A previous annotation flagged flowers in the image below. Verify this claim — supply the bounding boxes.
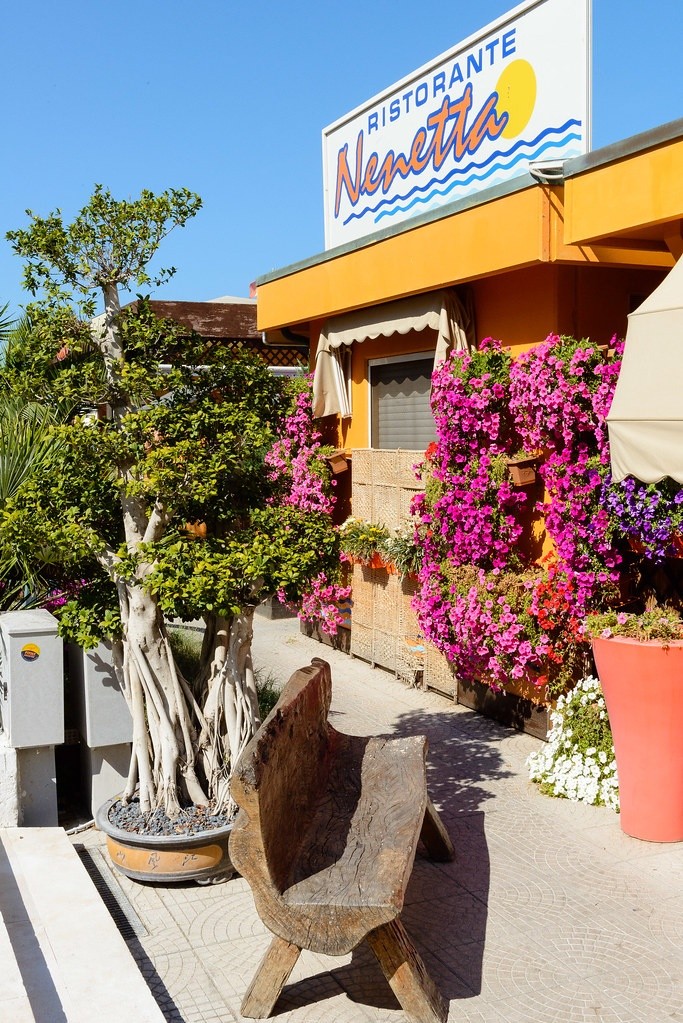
[339,515,389,564]
[411,332,683,691]
[262,366,352,636]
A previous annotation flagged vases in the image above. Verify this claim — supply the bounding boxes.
[587,632,682,842]
[356,553,386,569]
[506,454,537,485]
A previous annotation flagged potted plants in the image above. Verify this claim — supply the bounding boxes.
[2,186,342,887]
[384,529,421,582]
[320,444,348,474]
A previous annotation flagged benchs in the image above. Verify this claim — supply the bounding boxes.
[227,658,456,1023]
